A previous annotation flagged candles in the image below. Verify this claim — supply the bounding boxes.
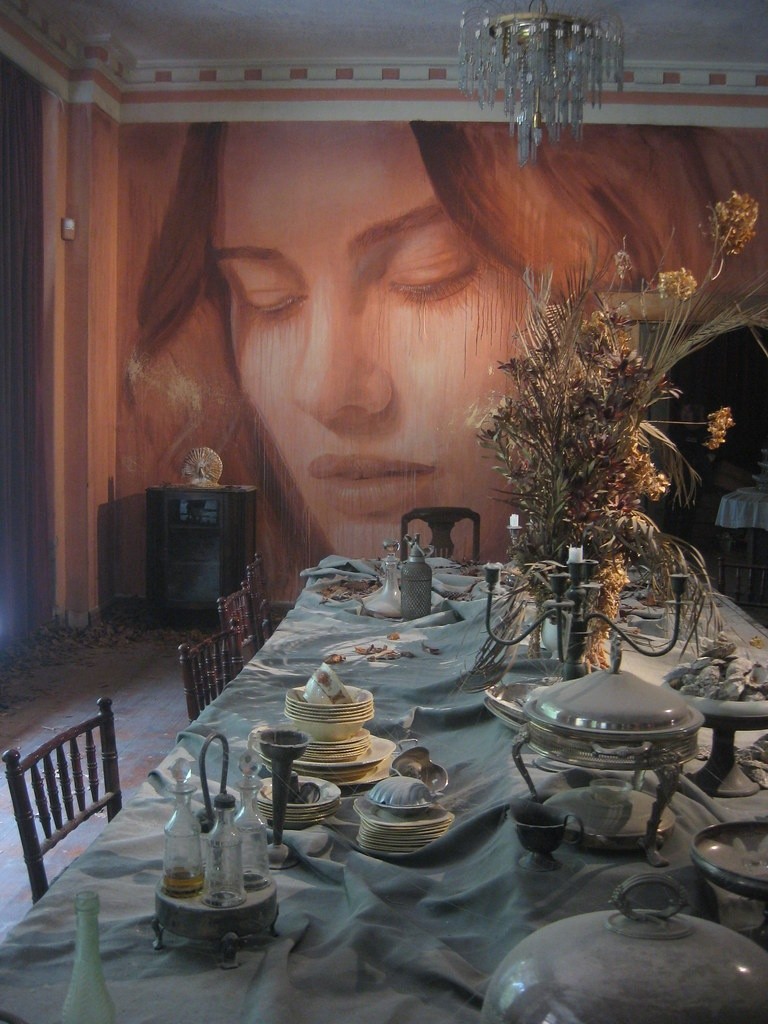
[510,514,519,528]
[567,543,584,562]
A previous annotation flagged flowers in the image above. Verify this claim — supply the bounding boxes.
[452,191,759,694]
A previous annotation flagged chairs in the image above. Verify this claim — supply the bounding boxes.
[717,556,768,630]
[216,580,259,671]
[1,696,122,906]
[399,507,481,565]
[178,618,244,723]
[246,550,273,654]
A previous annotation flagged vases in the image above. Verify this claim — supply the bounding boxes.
[542,608,573,660]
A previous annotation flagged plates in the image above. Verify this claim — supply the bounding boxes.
[352,798,455,854]
[236,728,396,787]
[256,774,341,830]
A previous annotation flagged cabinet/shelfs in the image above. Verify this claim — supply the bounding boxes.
[145,482,258,626]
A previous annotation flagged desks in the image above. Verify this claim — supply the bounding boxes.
[714,487,768,566]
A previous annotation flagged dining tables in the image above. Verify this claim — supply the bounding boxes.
[1,555,768,1024]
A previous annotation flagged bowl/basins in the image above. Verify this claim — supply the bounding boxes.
[282,686,375,741]
[590,776,633,805]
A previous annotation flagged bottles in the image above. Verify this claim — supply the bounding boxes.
[160,783,204,898]
[365,538,402,620]
[202,790,247,908]
[61,891,115,1024]
[401,533,432,618]
[233,775,271,893]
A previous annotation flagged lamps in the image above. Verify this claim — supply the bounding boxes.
[455,0,626,170]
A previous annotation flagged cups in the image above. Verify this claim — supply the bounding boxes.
[505,799,583,873]
